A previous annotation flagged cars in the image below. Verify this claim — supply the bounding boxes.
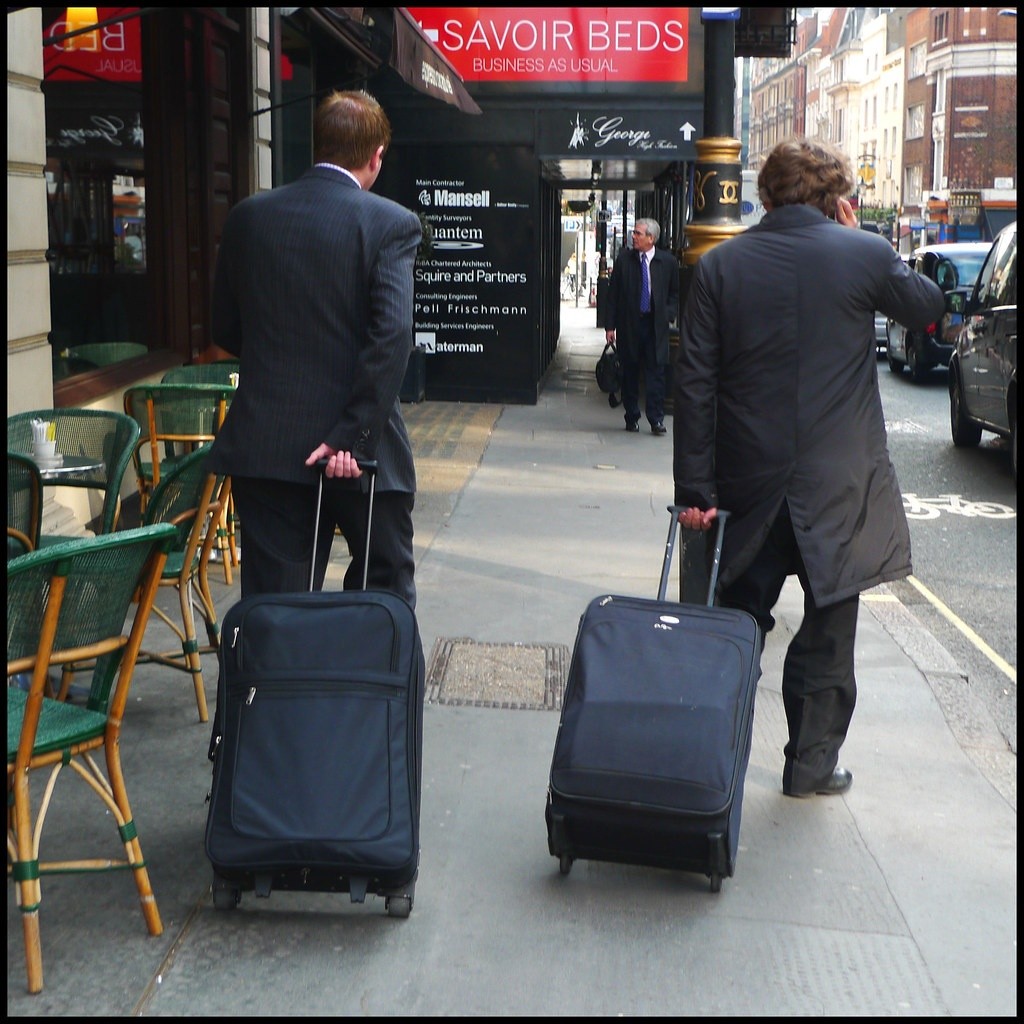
[874,243,994,377]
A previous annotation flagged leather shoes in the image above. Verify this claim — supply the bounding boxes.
[651,422,666,433]
[626,420,639,431]
[788,766,851,798]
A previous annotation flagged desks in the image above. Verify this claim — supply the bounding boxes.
[133,399,244,564]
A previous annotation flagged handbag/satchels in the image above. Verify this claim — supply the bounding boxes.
[596,342,625,409]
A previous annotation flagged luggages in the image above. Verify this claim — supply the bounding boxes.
[203,455,424,918]
[545,505,760,892]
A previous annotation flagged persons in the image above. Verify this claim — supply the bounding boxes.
[604,218,681,433]
[670,140,947,799]
[203,89,423,604]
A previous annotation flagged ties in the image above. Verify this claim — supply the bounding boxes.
[640,252,650,314]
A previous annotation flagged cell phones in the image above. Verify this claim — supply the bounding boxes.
[828,209,837,222]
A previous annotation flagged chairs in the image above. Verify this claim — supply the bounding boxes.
[7,407,140,553]
[5,450,59,701]
[159,358,241,406]
[55,443,221,724]
[0,522,178,994]
[123,383,239,586]
[62,342,147,371]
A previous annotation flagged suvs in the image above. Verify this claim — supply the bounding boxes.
[945,222,1017,480]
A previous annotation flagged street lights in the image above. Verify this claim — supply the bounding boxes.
[856,176,868,229]
